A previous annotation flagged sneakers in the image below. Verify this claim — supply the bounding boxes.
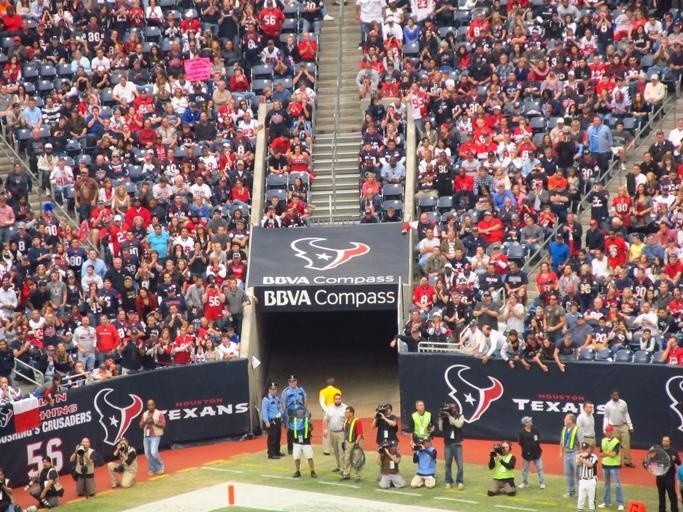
[563,491,578,498]
[519,484,528,488]
[598,503,611,509]
[333,468,360,483]
[540,484,546,488]
[445,481,463,491]
[292,472,300,477]
[311,470,316,479]
[269,452,285,459]
[617,506,623,510]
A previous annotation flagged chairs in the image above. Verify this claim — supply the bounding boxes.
[1,0,320,401]
[359,0,683,368]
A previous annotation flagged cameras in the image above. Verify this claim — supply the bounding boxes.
[120,442,126,452]
[77,445,85,457]
[41,498,53,509]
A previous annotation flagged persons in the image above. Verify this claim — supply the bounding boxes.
[261,377,364,482]
[642,435,681,511]
[107,439,137,488]
[0,1,334,404]
[1,438,96,511]
[356,1,681,371]
[372,400,464,489]
[139,399,166,475]
[488,416,546,496]
[559,392,635,510]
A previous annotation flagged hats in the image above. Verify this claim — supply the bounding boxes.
[521,417,531,423]
[287,375,296,380]
[580,442,589,448]
[605,425,613,431]
[267,382,277,390]
